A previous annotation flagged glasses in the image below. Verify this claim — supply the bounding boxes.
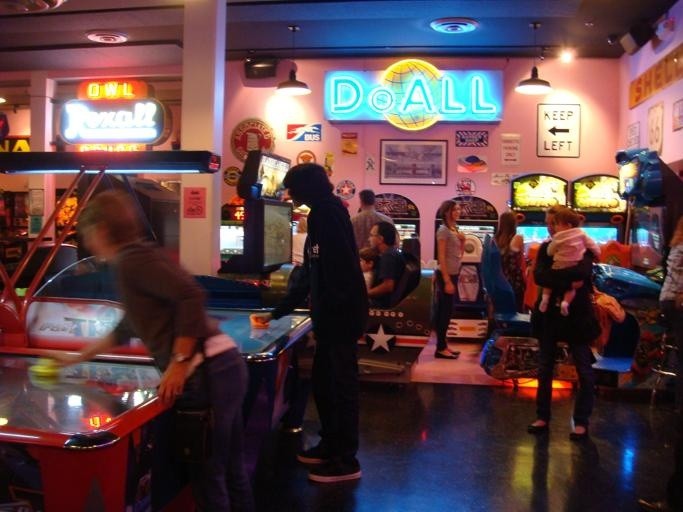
[369,234,378,237]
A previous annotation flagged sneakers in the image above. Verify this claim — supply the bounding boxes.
[298,439,322,464]
[309,458,361,482]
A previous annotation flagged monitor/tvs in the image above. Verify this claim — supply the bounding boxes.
[514,223,552,259]
[434,221,494,265]
[392,219,418,251]
[245,202,293,269]
[577,226,618,249]
[216,221,245,256]
[237,151,290,198]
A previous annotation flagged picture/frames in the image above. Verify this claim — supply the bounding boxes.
[380,139,447,186]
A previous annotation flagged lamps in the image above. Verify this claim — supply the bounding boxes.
[276,69,311,96]
[515,67,551,95]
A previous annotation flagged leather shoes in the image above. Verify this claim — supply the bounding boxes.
[570,426,589,439]
[528,422,548,432]
[450,351,460,354]
[435,351,458,359]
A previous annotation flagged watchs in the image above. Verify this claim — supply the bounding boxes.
[170,353,191,363]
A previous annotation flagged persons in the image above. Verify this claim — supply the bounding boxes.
[523,244,540,311]
[248,163,371,483]
[358,222,403,308]
[538,207,601,316]
[659,213,683,404]
[527,204,602,441]
[39,190,254,512]
[433,199,466,360]
[481,211,528,339]
[349,189,401,263]
